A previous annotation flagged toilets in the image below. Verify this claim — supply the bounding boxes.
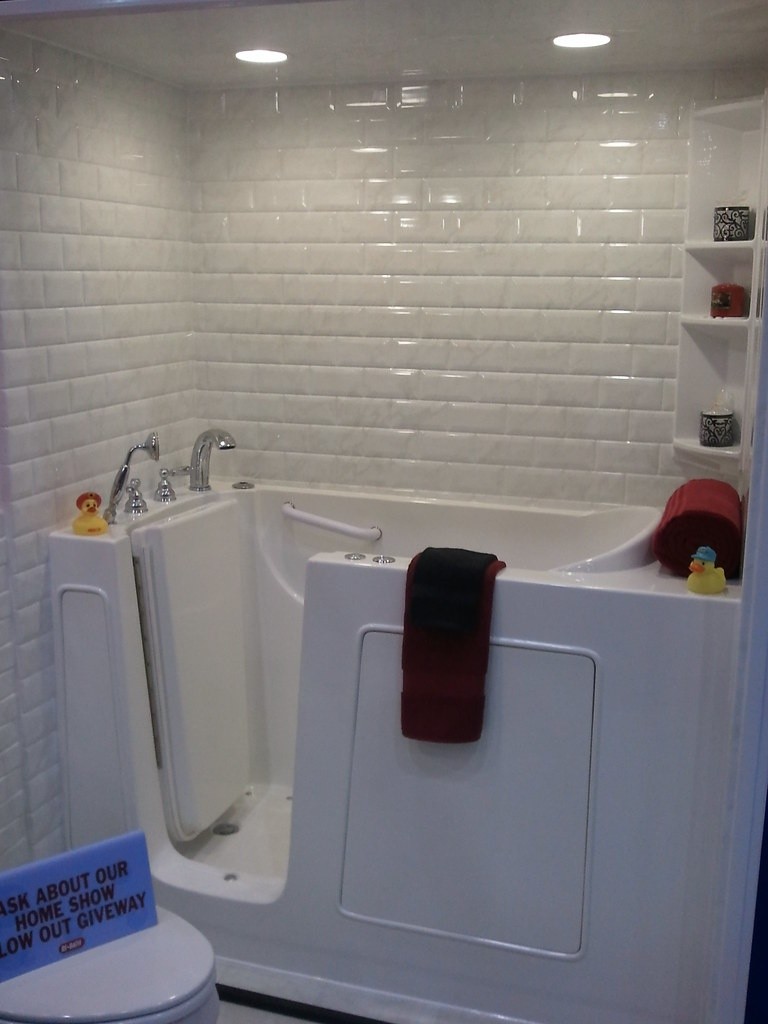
[0,904,219,1024]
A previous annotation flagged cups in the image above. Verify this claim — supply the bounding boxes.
[713,205,750,242]
[711,283,745,318]
[700,405,734,447]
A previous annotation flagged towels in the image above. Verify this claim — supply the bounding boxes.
[400,547,506,744]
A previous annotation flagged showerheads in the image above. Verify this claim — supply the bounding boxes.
[99,430,161,522]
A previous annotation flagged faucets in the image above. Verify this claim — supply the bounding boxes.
[189,429,236,491]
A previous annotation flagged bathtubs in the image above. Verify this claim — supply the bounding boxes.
[49,482,740,1024]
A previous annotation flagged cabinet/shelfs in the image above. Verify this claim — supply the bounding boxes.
[672,100,768,471]
